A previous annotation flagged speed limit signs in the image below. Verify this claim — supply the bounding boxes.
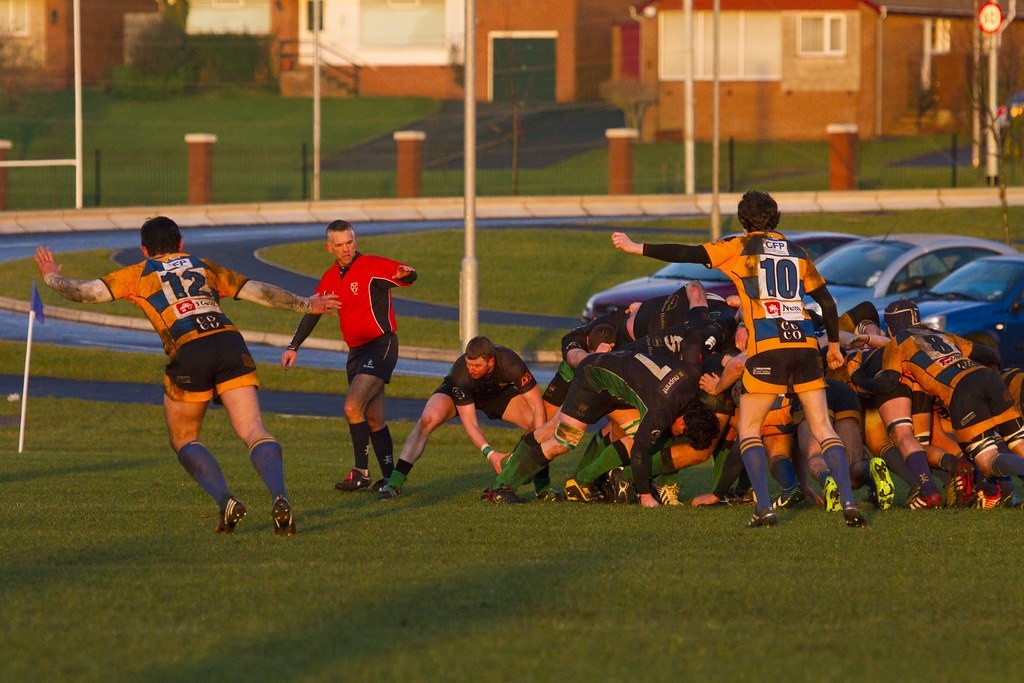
[976,1,1004,34]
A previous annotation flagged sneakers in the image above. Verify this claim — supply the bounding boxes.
[536,487,564,502]
[377,487,401,501]
[335,469,371,490]
[844,507,867,528]
[370,479,383,491]
[747,508,776,527]
[213,496,246,533]
[562,459,1022,510]
[272,496,295,536]
[493,488,520,505]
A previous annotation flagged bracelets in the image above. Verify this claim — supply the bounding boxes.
[480,443,494,459]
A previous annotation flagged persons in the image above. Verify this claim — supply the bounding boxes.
[377,337,566,503]
[612,188,866,528]
[281,221,417,491]
[487,281,1024,511]
[32,214,342,535]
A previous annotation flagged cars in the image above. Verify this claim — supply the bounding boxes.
[577,228,869,328]
[796,231,1024,332]
[880,253,1024,372]
